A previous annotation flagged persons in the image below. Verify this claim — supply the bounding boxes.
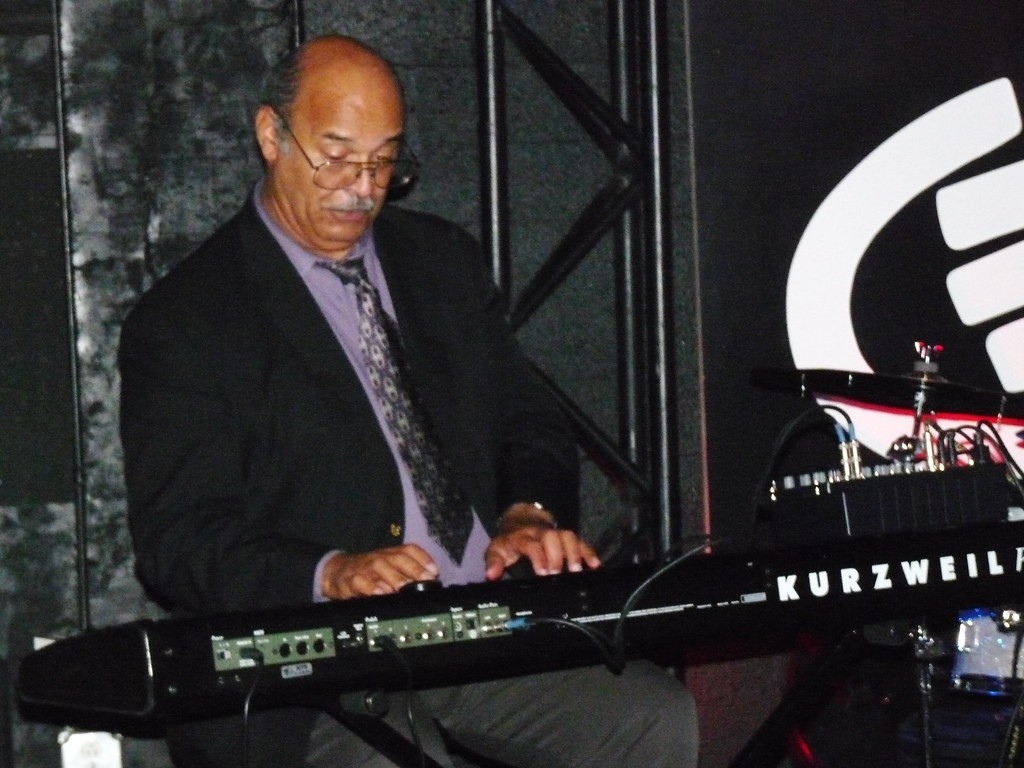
[118,33,700,768]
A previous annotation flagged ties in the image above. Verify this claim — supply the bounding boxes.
[317,255,474,566]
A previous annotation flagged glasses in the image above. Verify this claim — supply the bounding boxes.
[275,111,420,191]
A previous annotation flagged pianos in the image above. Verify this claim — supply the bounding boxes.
[10,517,1024,744]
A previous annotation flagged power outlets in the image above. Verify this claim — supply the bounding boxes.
[58,731,123,768]
